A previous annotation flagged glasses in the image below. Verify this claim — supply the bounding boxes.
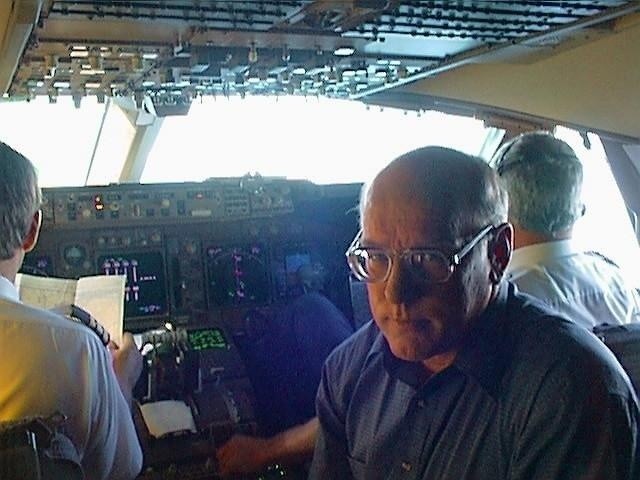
[345,223,496,287]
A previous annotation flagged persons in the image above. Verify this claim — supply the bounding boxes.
[211,126,639,478]
[0,140,148,480]
[304,143,638,479]
